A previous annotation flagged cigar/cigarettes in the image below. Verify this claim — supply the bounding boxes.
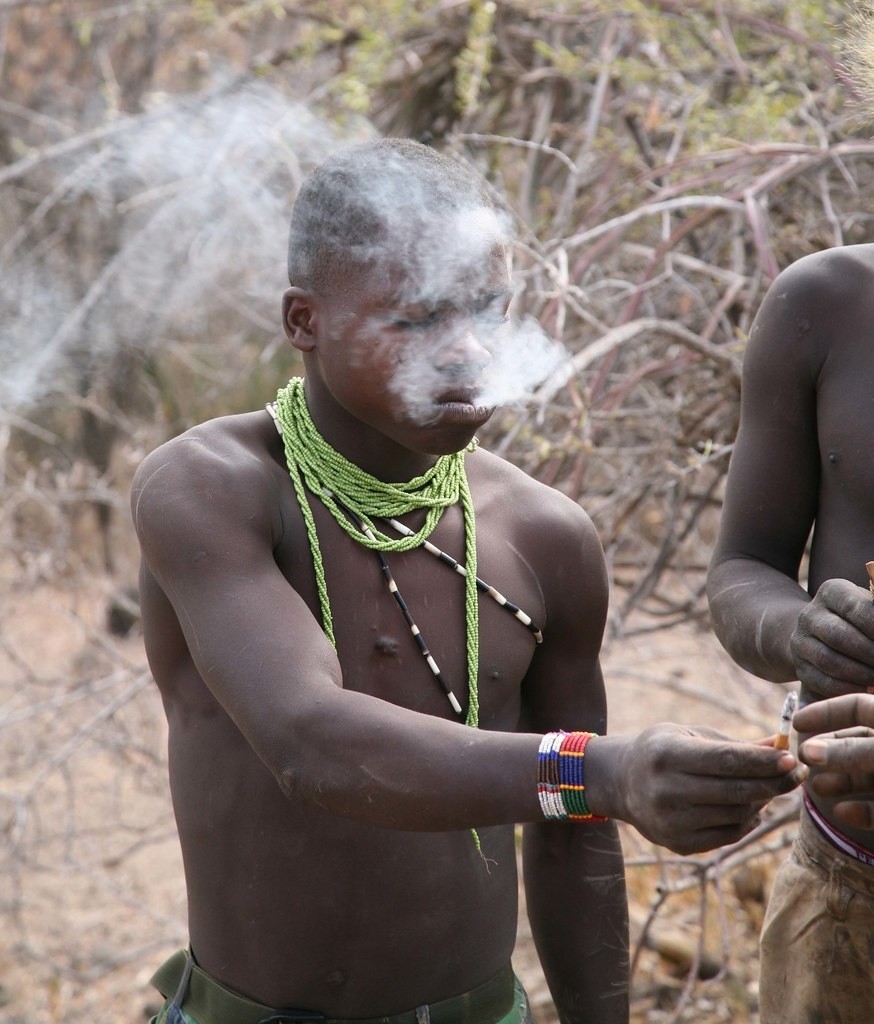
[773,692,797,749]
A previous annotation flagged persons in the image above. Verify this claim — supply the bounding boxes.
[129,138,810,1024]
[793,693,874,830]
[707,241,874,1024]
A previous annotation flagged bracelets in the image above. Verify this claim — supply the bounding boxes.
[537,731,608,822]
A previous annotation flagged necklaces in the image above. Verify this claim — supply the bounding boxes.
[264,376,544,728]
